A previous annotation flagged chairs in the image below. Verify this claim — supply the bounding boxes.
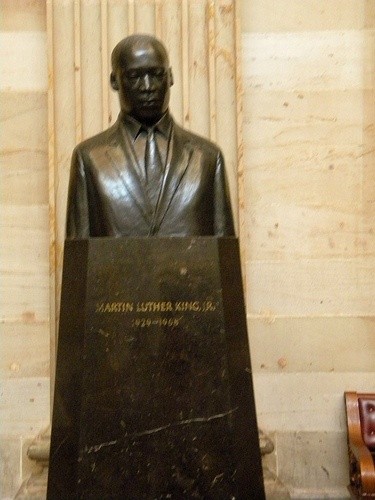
[344,390,375,500]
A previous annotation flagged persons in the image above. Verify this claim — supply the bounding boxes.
[63,35,234,240]
